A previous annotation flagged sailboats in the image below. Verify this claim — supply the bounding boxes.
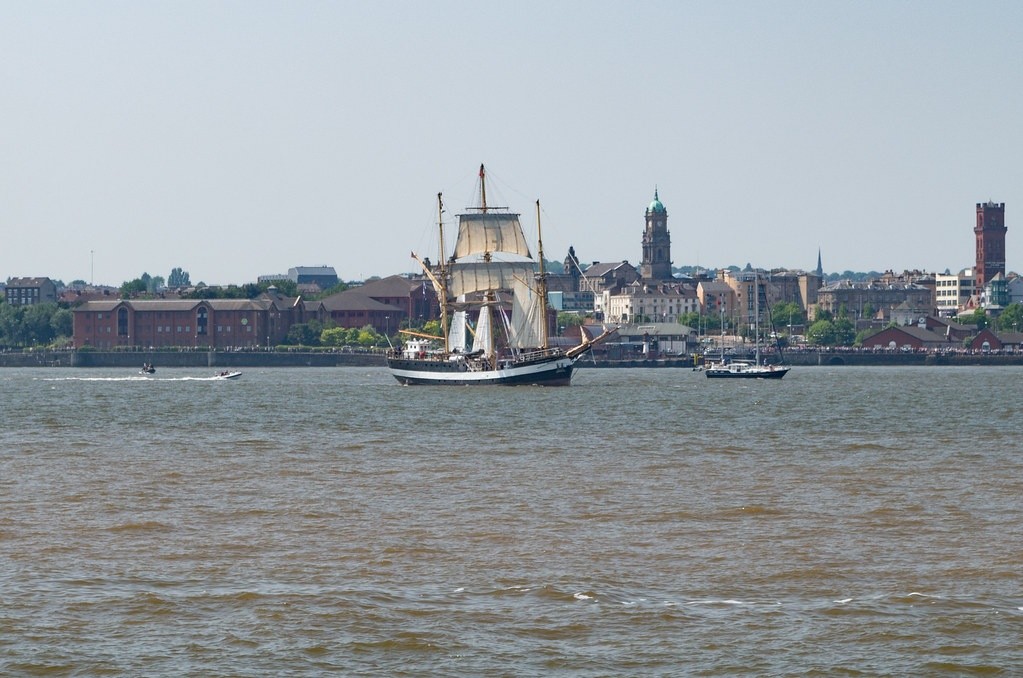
[705,268,793,381]
[382,163,624,387]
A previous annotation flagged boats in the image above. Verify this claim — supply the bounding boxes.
[138,365,155,374]
[219,371,243,380]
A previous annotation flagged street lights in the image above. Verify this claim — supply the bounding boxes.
[266,336,270,347]
[852,310,857,332]
[385,317,390,336]
[194,335,198,348]
